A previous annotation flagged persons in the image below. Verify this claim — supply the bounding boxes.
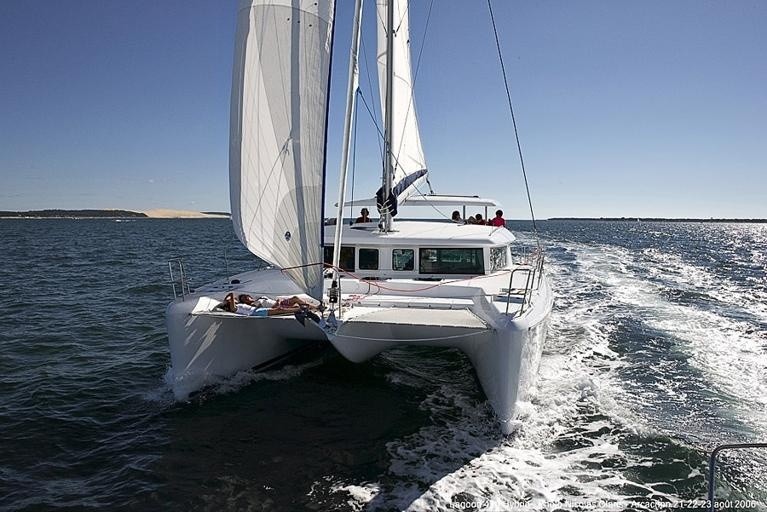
[476,214,485,225]
[219,292,307,316]
[355,208,372,223]
[451,211,459,223]
[490,210,505,226]
[468,216,475,224]
[238,292,322,310]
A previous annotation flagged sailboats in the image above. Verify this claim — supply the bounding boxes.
[165,0,554,437]
